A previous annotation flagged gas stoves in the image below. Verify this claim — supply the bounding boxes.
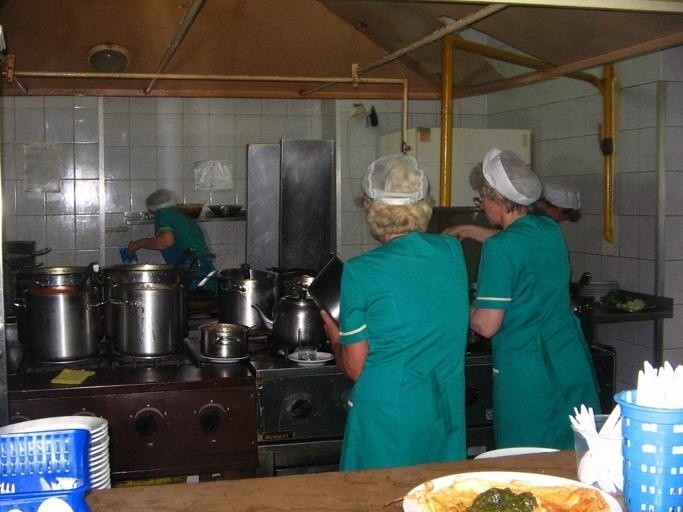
[14,341,197,369]
[247,331,295,361]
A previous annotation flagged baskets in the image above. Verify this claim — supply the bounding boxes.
[0,429,90,512]
[613,387,683,512]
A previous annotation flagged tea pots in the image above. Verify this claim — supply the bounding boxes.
[250,289,326,346]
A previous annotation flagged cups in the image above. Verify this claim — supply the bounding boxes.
[296,329,319,360]
[571,414,626,495]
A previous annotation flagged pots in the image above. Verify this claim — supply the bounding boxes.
[276,270,320,293]
[103,264,191,358]
[11,265,100,360]
[1,240,52,315]
[197,322,249,356]
[200,264,275,332]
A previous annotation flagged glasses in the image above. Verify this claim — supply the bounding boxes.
[472,190,493,208]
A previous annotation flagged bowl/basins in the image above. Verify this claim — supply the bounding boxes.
[207,205,242,216]
[172,202,203,218]
[306,255,346,322]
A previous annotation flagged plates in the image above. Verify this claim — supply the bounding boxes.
[287,350,334,367]
[0,414,113,491]
[401,470,623,512]
[572,279,619,302]
[198,354,249,363]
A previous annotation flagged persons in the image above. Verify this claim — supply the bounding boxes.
[534,182,582,223]
[125,187,219,297]
[318,151,471,472]
[438,146,605,452]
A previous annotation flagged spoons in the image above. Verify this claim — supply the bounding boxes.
[568,404,625,493]
